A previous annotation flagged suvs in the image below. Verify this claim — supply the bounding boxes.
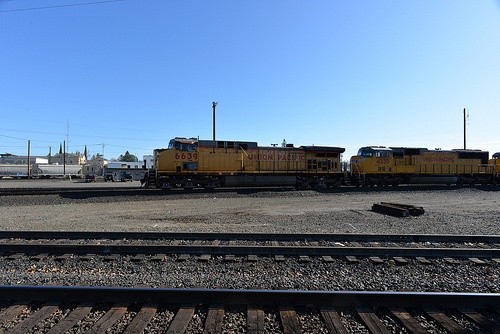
[120,172,133,181]
[87,174,97,182]
[104,173,115,182]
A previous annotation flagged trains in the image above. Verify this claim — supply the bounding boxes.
[153,137,500,192]
